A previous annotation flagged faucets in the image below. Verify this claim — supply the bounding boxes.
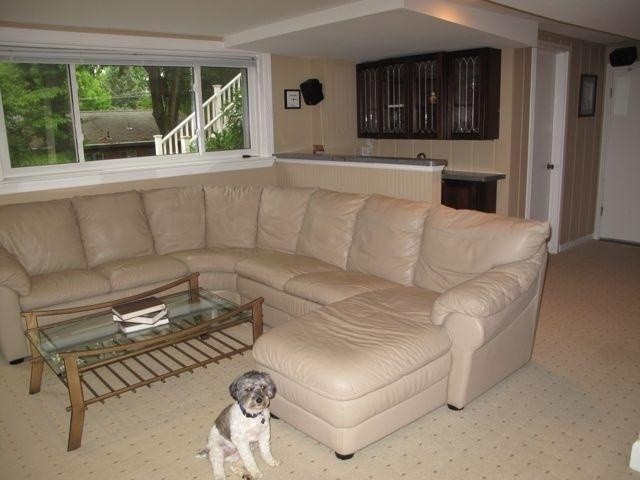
[417,152,427,160]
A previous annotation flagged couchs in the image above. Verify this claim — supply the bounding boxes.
[0,183,548,460]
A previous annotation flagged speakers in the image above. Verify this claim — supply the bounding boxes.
[609,47,636,66]
[300,78,324,105]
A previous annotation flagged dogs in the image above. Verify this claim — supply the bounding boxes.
[196,369,281,479]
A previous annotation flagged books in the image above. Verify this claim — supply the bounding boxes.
[112,307,168,325]
[111,295,166,321]
[115,315,169,334]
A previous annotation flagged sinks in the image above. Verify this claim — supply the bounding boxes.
[392,159,442,166]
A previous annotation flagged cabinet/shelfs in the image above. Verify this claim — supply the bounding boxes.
[356,55,410,139]
[410,47,501,140]
[441,180,497,214]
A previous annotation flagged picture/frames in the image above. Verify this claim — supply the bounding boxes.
[577,73,598,119]
[284,89,302,109]
